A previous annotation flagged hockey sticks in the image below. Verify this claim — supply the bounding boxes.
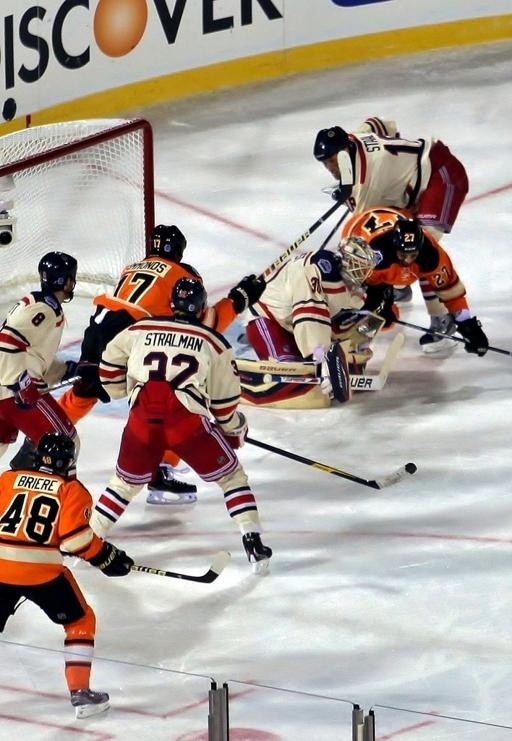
[209,423,416,489]
[60,550,231,583]
[255,150,353,284]
[239,332,406,391]
[238,208,350,343]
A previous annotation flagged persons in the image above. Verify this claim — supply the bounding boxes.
[246,249,396,409]
[341,207,488,355]
[88,279,271,561]
[0,432,132,707]
[0,251,97,461]
[9,225,265,471]
[313,116,470,346]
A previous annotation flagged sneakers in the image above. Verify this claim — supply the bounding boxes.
[392,285,412,302]
[419,313,455,345]
[71,688,110,706]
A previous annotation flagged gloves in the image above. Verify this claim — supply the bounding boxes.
[7,369,40,410]
[89,541,134,576]
[228,273,266,313]
[214,412,248,450]
[61,360,80,381]
[456,315,488,356]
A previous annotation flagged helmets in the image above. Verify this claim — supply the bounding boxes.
[148,225,186,264]
[335,236,383,291]
[168,277,208,323]
[313,126,349,161]
[38,252,77,292]
[38,432,75,477]
[391,220,425,253]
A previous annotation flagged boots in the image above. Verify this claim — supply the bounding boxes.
[242,532,272,562]
[147,465,197,493]
